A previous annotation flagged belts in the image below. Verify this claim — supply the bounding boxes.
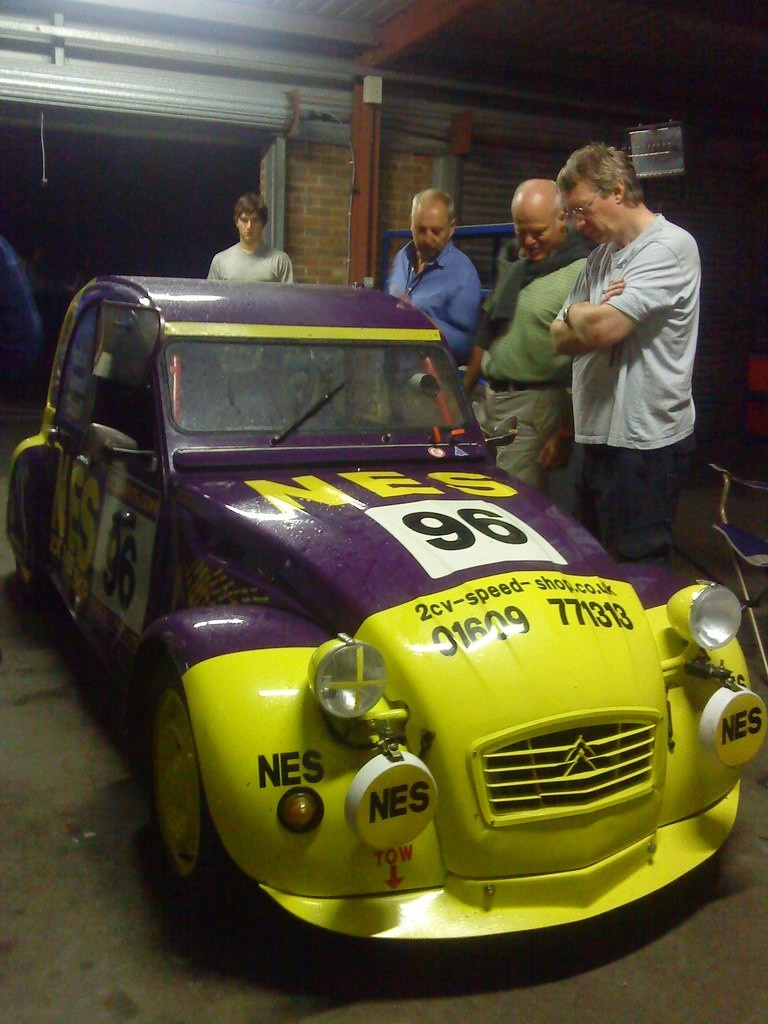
[484,377,548,392]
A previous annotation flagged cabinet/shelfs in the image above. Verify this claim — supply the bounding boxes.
[383,223,515,388]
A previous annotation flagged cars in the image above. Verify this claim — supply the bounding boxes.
[4,274,768,946]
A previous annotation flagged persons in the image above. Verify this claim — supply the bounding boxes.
[388,188,481,385]
[462,179,588,491]
[207,193,296,283]
[550,143,702,572]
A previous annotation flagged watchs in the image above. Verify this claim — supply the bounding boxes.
[563,304,574,329]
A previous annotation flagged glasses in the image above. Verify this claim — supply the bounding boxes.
[560,190,601,223]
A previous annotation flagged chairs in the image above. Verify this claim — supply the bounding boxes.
[709,460,768,683]
